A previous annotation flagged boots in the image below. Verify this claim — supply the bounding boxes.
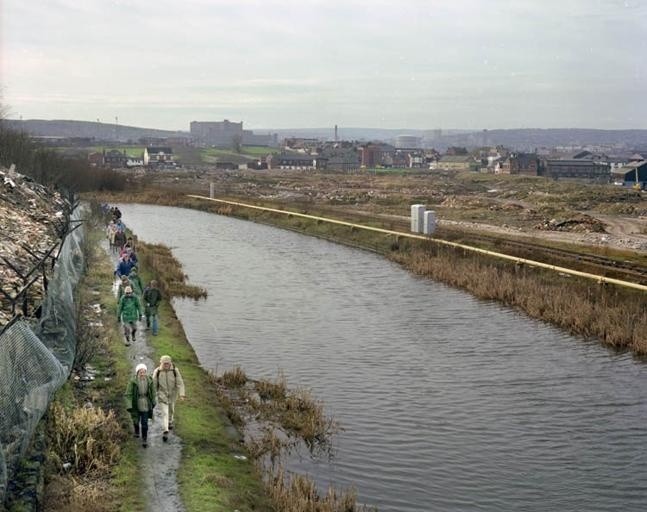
[133,423,140,438]
[141,424,148,447]
[125,331,135,346]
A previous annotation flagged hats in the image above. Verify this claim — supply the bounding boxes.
[135,363,147,375]
[160,354,171,363]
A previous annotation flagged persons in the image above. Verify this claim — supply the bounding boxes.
[151,354,184,444]
[97,201,137,279]
[142,279,160,337]
[116,285,141,349]
[125,362,153,449]
[117,274,139,304]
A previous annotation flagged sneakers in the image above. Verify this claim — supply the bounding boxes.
[168,421,172,430]
[163,431,169,441]
[145,325,158,335]
